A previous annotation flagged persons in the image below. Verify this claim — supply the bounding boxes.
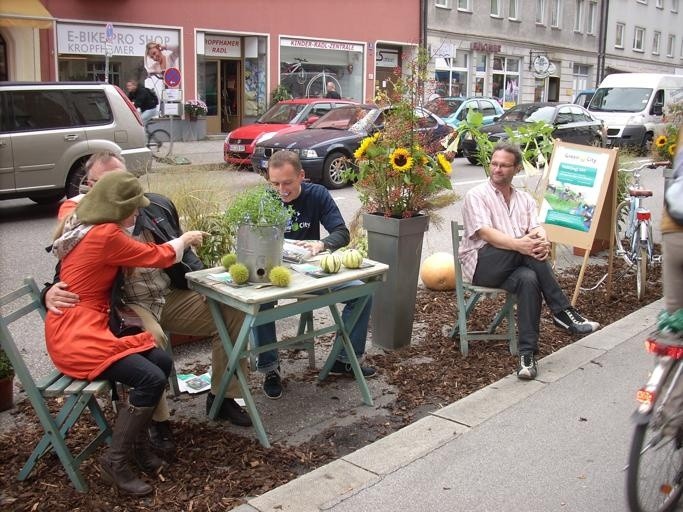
[42,169,212,496]
[35,150,253,454]
[141,41,180,74]
[249,149,377,400]
[125,79,159,124]
[659,125,683,439]
[325,81,342,99]
[455,141,602,380]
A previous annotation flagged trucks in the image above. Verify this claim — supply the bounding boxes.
[588,72,682,155]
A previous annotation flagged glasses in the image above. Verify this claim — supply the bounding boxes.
[488,161,515,170]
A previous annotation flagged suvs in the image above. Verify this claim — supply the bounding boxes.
[425,97,506,128]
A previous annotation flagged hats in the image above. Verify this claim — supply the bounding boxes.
[74,170,150,224]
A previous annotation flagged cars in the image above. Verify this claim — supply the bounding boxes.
[224,98,360,167]
[460,100,608,167]
[252,103,455,187]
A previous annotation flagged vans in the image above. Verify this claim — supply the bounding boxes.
[572,88,599,109]
[0,81,153,208]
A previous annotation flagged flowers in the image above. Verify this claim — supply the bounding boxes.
[654,101,683,168]
[339,40,460,221]
[227,181,299,242]
[184,99,208,115]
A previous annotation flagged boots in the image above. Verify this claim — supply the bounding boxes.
[129,414,163,475]
[100,397,153,496]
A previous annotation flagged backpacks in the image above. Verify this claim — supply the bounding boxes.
[662,147,683,219]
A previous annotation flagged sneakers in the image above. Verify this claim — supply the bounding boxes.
[263,365,282,401]
[328,359,376,378]
[552,307,599,334]
[516,348,538,378]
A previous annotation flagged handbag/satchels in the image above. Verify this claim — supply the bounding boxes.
[107,302,144,339]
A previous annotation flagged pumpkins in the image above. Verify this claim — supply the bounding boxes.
[320,255,341,273]
[342,249,362,268]
[420,252,457,290]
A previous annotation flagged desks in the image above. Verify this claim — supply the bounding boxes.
[185,251,390,450]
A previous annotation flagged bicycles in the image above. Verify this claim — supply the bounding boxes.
[616,159,673,301]
[142,118,173,161]
[281,58,343,98]
[626,306,683,511]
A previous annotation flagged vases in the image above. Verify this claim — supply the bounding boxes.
[236,219,284,283]
[363,212,430,351]
[190,113,198,121]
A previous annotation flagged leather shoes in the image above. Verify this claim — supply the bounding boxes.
[205,392,251,425]
[148,416,176,452]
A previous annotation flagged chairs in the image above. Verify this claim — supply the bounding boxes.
[249,302,315,370]
[0,277,115,495]
[109,331,180,414]
[448,220,518,358]
